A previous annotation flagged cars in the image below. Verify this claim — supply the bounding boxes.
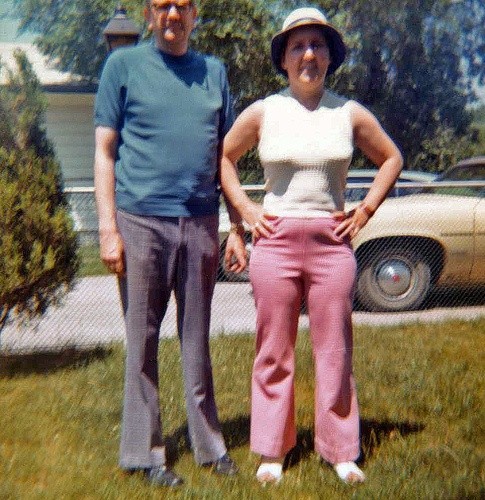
[219,167,444,285]
[345,156,485,314]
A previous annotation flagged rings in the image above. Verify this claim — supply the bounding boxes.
[231,225,245,235]
[255,222,260,228]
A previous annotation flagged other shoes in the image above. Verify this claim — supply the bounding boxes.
[203,453,238,477]
[256,454,285,484]
[138,466,182,487]
[334,459,366,486]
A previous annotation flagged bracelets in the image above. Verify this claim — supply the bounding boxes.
[360,201,374,218]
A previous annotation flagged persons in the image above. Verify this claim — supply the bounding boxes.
[221,6,403,487]
[95,0,249,489]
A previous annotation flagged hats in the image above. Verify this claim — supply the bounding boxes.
[271,7,347,76]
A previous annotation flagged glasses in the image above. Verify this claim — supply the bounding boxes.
[150,2,194,16]
